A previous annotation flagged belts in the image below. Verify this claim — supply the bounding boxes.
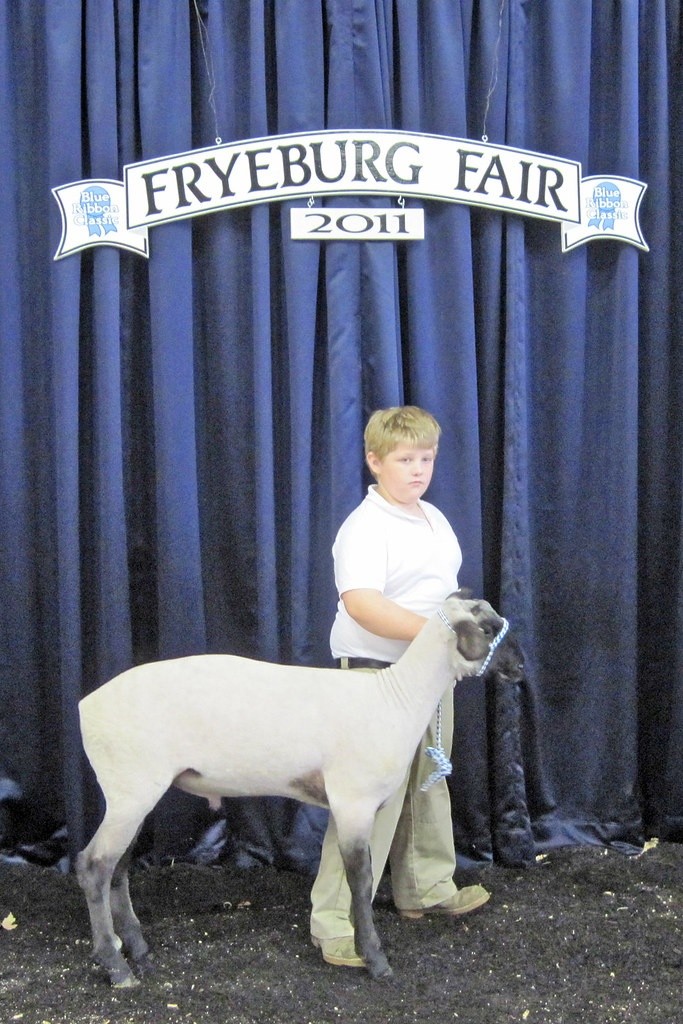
[335,657,392,669]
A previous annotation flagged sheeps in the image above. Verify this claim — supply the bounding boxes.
[73,590,530,988]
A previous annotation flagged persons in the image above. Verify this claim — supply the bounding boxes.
[311,406,489,968]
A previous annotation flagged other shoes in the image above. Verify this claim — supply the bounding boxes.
[403,885,489,919]
[311,927,366,968]
